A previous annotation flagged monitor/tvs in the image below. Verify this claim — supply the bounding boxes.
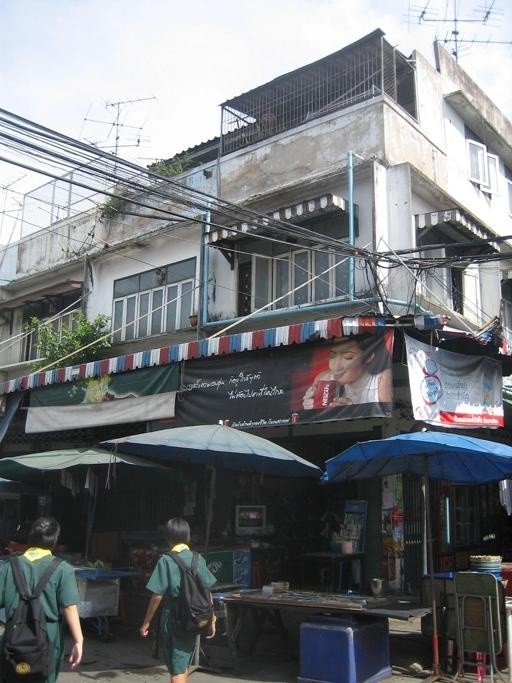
[235,504,267,535]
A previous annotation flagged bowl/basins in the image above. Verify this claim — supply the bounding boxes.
[469,558,503,582]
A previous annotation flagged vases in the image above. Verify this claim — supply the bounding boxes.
[189,315,197,326]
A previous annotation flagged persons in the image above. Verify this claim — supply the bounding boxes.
[0,511,86,682]
[301,333,393,411]
[136,515,218,682]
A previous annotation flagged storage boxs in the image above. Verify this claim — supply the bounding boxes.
[296,614,394,683]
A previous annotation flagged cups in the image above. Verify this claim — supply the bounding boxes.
[369,578,384,595]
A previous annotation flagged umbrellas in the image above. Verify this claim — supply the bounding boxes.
[0,444,179,565]
[324,424,512,673]
[100,418,326,553]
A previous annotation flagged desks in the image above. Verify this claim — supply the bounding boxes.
[0,534,512,675]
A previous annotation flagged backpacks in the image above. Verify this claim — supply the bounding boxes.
[1,557,63,681]
[161,551,212,637]
